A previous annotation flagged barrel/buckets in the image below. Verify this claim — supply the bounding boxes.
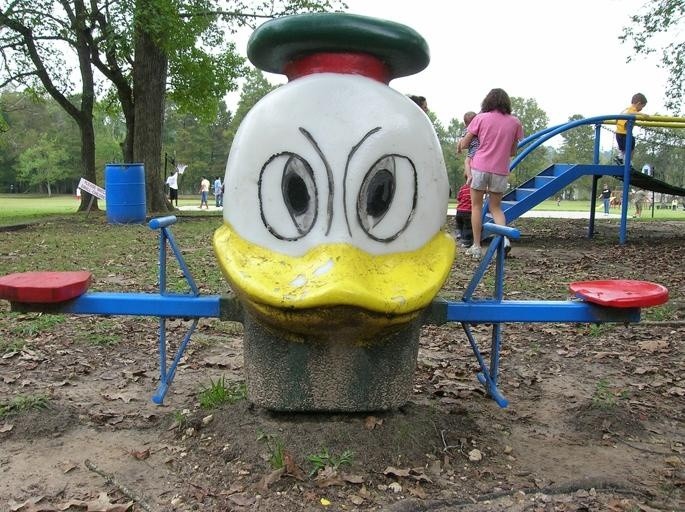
[104,163,147,225]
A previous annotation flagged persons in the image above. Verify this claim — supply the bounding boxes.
[214,177,224,207]
[409,96,428,113]
[457,111,481,185]
[198,176,210,209]
[460,88,525,260]
[633,189,648,219]
[557,197,560,206]
[598,183,613,216]
[77,188,82,200]
[166,172,178,207]
[457,183,473,247]
[671,197,678,210]
[613,93,648,167]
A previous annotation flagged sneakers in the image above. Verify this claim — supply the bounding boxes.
[614,156,623,165]
[503,239,511,259]
[465,245,482,256]
